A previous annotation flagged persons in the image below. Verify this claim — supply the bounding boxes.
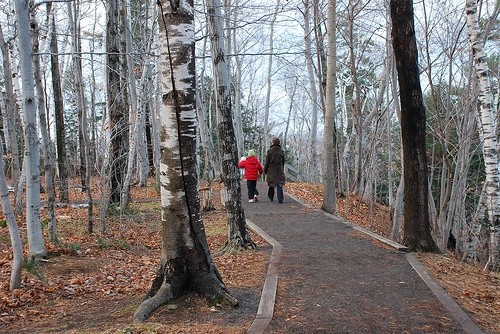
[238,150,264,203]
[264,136,285,204]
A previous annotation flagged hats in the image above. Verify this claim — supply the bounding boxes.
[248,150,255,157]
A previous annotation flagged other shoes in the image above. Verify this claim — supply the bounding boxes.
[254,195,258,202]
[249,199,255,203]
[279,201,283,203]
